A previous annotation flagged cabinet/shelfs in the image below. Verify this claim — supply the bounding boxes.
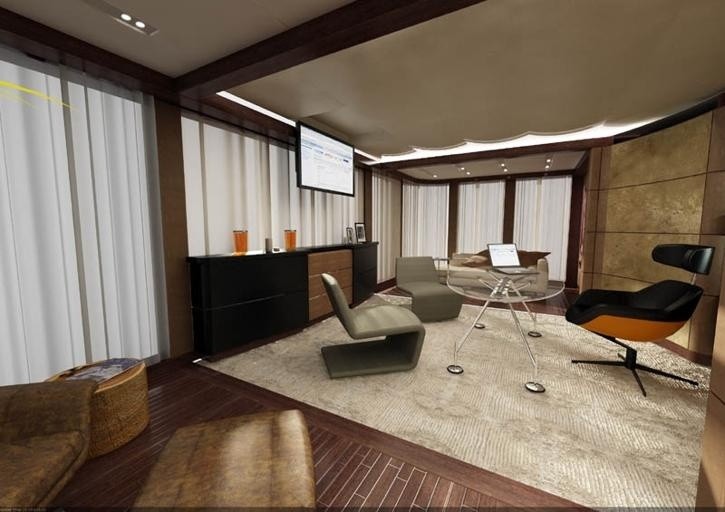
[184,241,380,363]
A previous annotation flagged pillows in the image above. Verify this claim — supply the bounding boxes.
[462,249,551,269]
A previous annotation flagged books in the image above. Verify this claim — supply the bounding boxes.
[66,358,145,385]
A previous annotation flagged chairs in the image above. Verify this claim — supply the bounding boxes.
[315,271,425,380]
[394,256,464,323]
[564,244,715,397]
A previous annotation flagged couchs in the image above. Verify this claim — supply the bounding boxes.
[447,252,549,296]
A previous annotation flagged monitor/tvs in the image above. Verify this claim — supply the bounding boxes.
[295,121,355,198]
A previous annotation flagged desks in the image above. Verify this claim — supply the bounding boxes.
[446,267,565,377]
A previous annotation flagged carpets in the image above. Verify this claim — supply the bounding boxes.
[192,291,712,512]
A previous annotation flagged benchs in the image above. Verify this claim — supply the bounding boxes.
[0,380,98,511]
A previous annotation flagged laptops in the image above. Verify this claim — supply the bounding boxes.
[487,243,539,275]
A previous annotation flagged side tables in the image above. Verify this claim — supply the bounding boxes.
[43,357,149,460]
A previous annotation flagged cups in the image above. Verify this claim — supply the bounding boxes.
[284,229,297,252]
[232,230,248,256]
[266,238,273,256]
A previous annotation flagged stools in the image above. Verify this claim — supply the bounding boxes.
[131,408,316,512]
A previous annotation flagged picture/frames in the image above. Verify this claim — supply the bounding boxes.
[354,222,367,243]
